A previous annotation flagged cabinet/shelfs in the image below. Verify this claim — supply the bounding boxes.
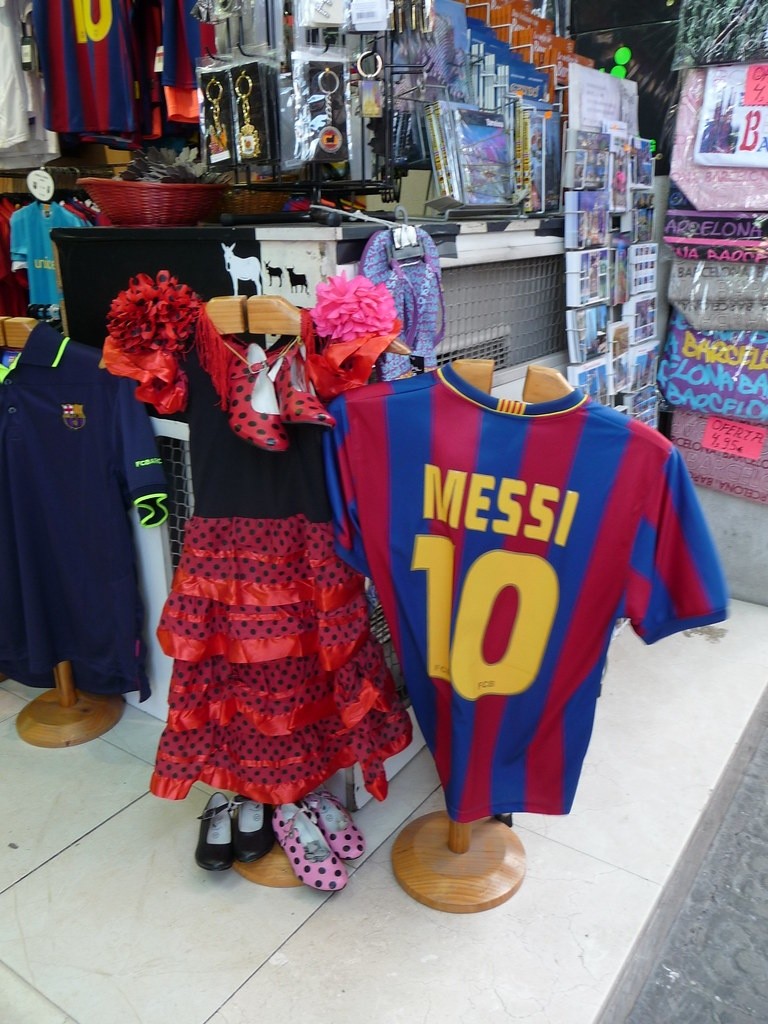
[52,206,568,812]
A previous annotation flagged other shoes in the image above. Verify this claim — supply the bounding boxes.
[226,343,289,453]
[307,791,365,860]
[267,348,336,429]
[272,802,348,892]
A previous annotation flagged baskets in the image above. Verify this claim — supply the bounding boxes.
[224,174,299,215]
[76,177,227,227]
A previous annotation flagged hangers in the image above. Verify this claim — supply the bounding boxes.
[0,164,113,222]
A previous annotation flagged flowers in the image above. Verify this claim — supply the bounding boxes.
[106,269,203,355]
[310,267,398,342]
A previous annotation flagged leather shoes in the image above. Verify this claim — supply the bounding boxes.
[234,794,275,863]
[195,792,234,872]
